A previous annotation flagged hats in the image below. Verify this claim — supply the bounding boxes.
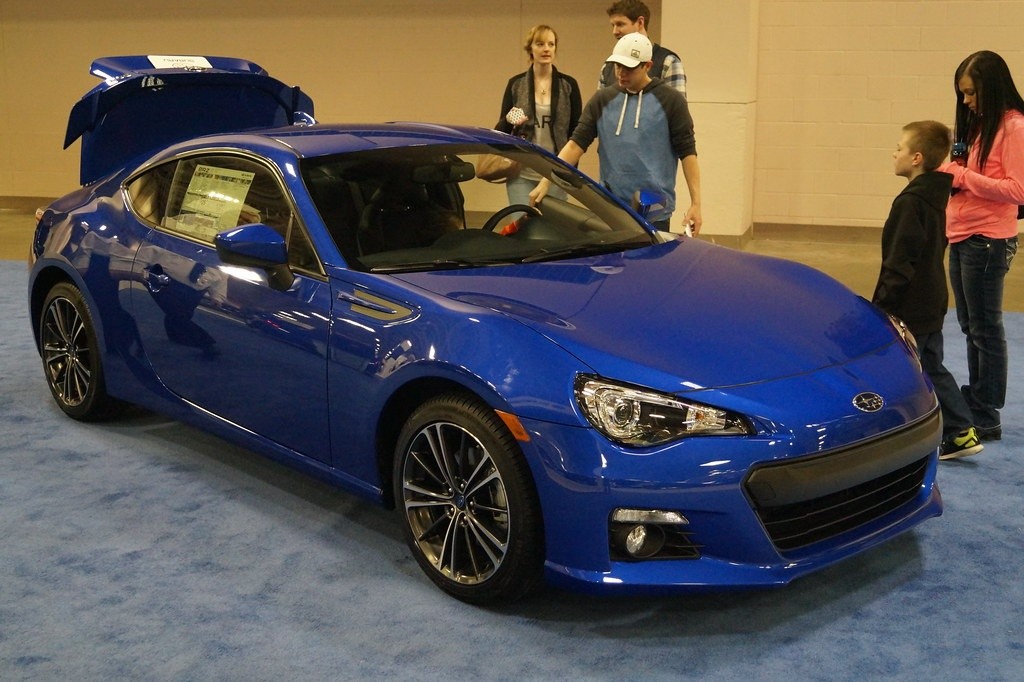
[605,31,652,68]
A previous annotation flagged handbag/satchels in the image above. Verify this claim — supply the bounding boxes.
[475,123,521,183]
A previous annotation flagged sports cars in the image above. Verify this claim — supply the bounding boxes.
[25,52,946,608]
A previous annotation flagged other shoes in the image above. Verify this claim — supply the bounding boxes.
[973,422,1002,440]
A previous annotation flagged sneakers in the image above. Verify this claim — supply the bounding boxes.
[937,425,985,461]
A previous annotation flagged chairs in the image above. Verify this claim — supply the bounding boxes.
[356,167,462,256]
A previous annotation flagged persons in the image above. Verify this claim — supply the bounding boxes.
[529,32,702,238]
[494,25,582,221]
[871,121,983,459]
[937,51,1024,441]
[597,0,687,101]
